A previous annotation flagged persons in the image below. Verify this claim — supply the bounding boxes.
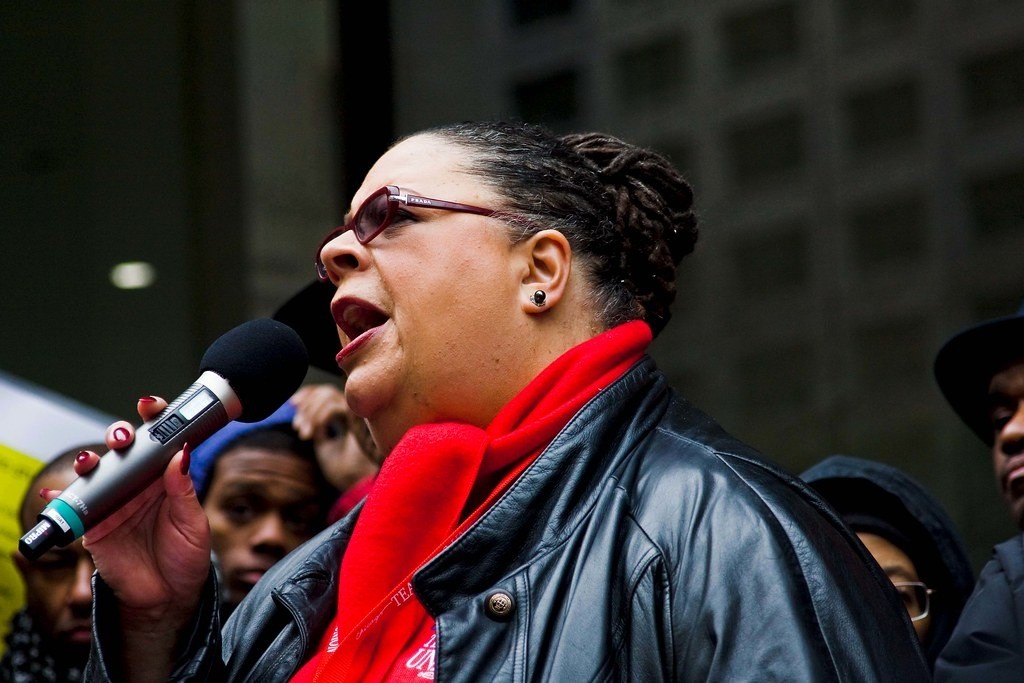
[38,117,941,683]
[0,308,1024,683]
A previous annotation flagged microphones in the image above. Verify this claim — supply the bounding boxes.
[18,315,308,560]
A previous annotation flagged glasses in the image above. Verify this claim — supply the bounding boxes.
[895,581,933,624]
[314,184,548,281]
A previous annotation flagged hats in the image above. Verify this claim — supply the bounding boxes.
[932,318,1024,451]
